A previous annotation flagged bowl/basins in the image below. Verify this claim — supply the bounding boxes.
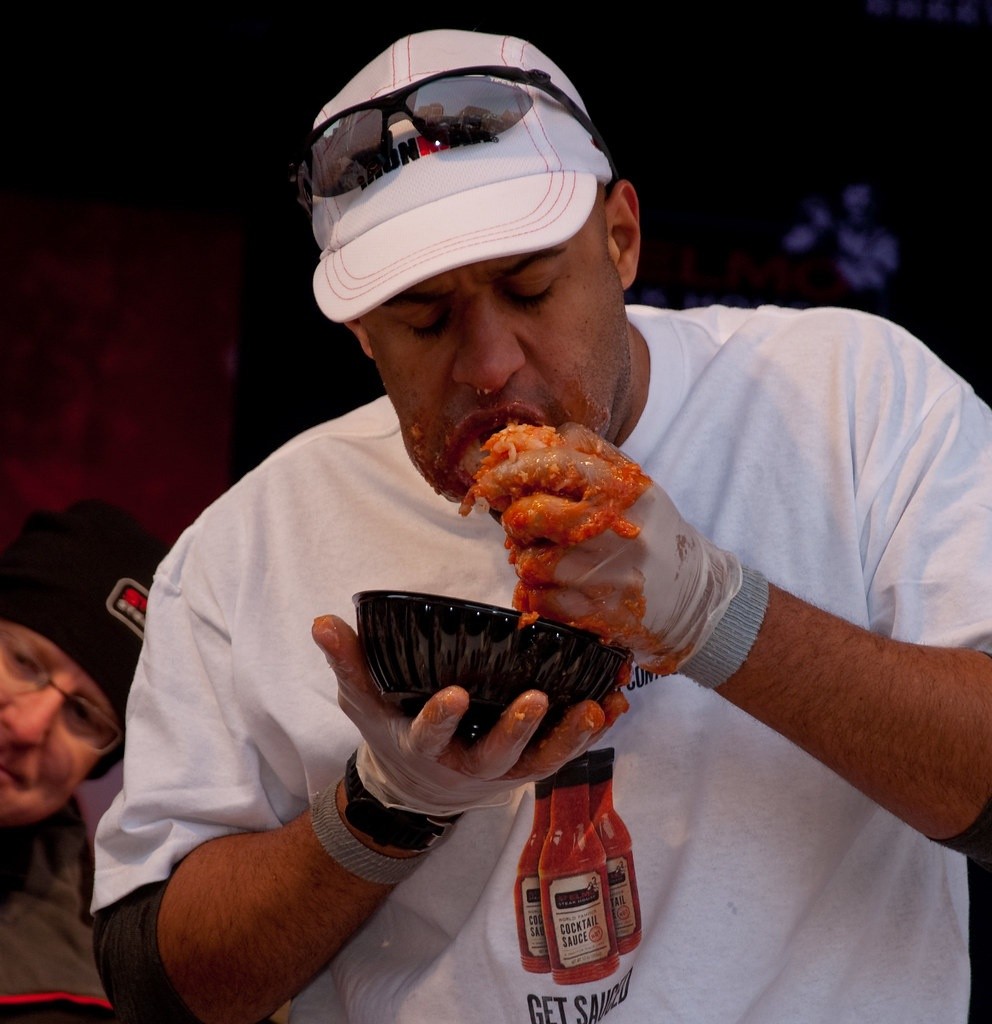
[352,590,636,750]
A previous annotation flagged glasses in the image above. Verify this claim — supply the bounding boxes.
[0,627,122,754]
[289,64,619,237]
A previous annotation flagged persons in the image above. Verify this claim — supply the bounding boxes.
[90,32,991,1024]
[1,497,166,1024]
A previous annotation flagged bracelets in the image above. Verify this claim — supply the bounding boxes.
[311,777,432,884]
[676,565,768,689]
[344,748,463,851]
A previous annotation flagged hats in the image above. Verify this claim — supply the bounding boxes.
[313,27,611,323]
[0,499,174,779]
